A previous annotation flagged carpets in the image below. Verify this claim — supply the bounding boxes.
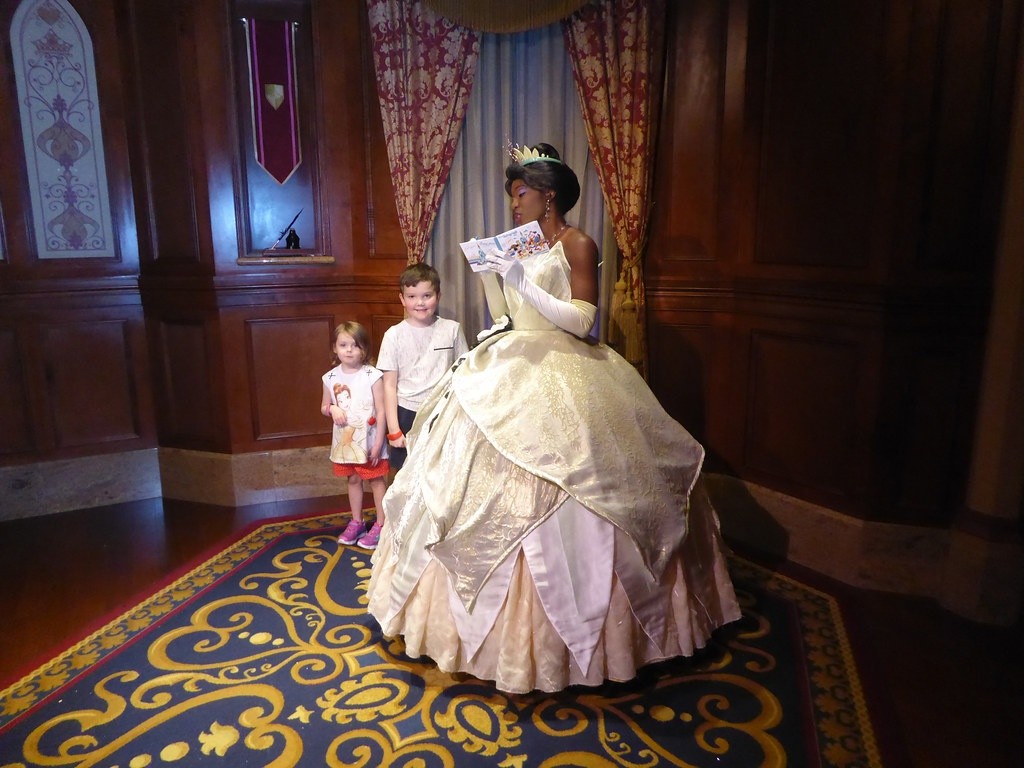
[0,503,910,768]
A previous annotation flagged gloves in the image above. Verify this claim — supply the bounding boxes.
[485,249,598,339]
[469,237,510,322]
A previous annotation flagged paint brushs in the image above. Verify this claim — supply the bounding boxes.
[271,208,304,249]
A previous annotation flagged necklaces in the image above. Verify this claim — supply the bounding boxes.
[548,222,568,245]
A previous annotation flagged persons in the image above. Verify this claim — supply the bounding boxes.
[320,321,390,550]
[365,138,743,694]
[377,263,468,469]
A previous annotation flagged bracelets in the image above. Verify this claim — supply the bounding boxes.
[386,431,402,441]
[326,402,334,417]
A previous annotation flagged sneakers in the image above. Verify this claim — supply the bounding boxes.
[338,520,367,545]
[357,522,383,549]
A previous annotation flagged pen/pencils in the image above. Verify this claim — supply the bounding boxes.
[474,235,479,240]
